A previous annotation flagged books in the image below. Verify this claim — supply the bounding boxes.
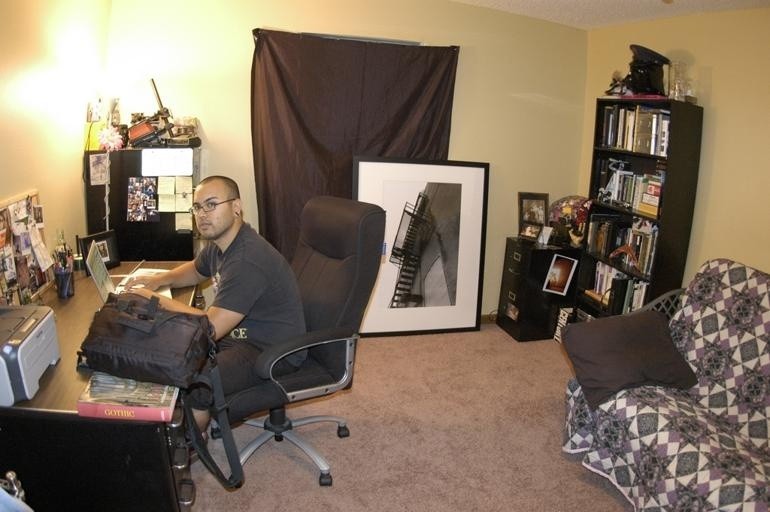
[586,104,670,316]
[77,372,179,422]
[553,308,600,345]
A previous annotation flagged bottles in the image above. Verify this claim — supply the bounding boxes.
[668,63,687,102]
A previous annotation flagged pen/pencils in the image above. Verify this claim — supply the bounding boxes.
[129,259,145,274]
[52,243,73,299]
[76,235,79,257]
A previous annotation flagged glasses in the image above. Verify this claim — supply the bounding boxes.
[189,199,236,217]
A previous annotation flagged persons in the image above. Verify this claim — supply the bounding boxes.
[124,176,308,465]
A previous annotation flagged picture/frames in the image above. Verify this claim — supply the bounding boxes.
[79,229,121,275]
[350,154,489,337]
[518,191,549,241]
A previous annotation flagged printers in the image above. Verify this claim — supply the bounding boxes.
[0,305,61,407]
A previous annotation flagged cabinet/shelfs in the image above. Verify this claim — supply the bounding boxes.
[494,238,567,340]
[571,97,703,331]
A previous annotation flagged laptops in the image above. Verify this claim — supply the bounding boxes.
[85,239,173,308]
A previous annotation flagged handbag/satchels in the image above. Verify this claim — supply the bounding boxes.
[79,292,212,390]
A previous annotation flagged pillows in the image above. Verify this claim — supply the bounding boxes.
[558,309,699,412]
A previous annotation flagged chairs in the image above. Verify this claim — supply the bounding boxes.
[208,194,386,490]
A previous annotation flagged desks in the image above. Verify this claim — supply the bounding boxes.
[1,260,202,511]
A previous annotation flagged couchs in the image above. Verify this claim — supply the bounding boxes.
[561,257,769,512]
